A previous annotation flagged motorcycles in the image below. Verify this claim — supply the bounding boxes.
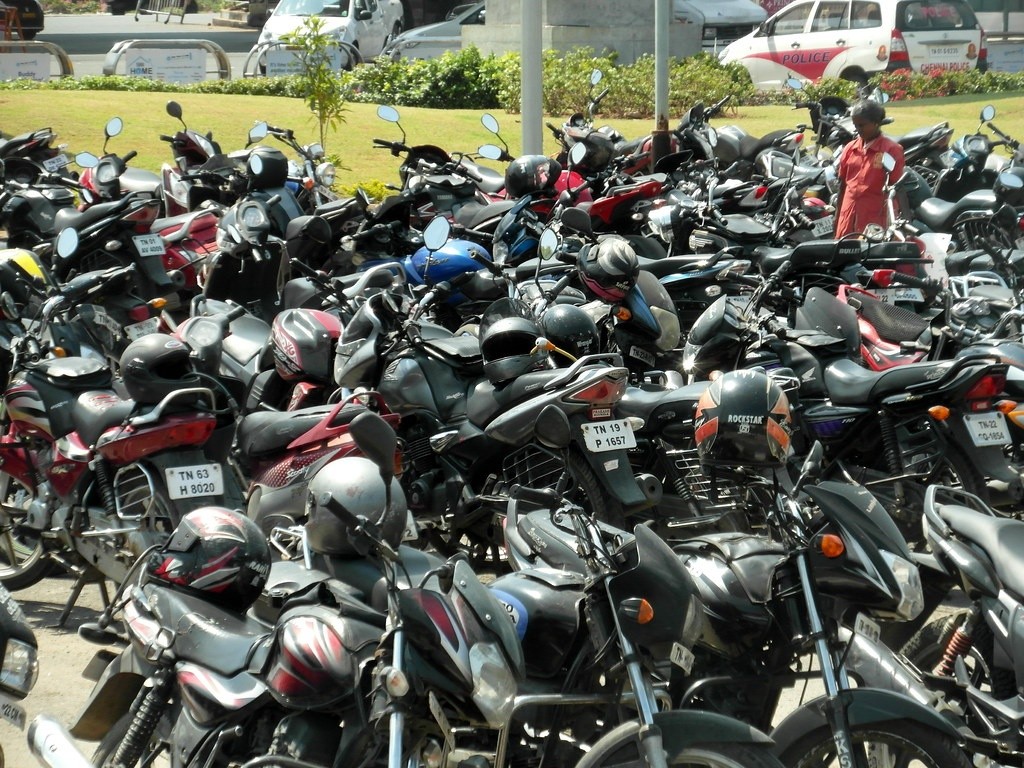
[0,84,1024,768]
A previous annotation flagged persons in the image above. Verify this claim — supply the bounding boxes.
[179,0,198,14]
[111,0,150,15]
[832,99,912,240]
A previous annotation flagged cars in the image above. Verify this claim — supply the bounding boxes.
[0,0,45,40]
[685,0,770,54]
[717,0,988,102]
[379,0,485,70]
[257,0,406,77]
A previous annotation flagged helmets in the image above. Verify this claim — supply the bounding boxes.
[544,304,599,367]
[247,145,288,188]
[479,297,549,387]
[120,333,201,405]
[575,130,614,173]
[505,155,562,199]
[148,505,272,613]
[305,457,408,557]
[695,369,792,467]
[577,238,640,302]
